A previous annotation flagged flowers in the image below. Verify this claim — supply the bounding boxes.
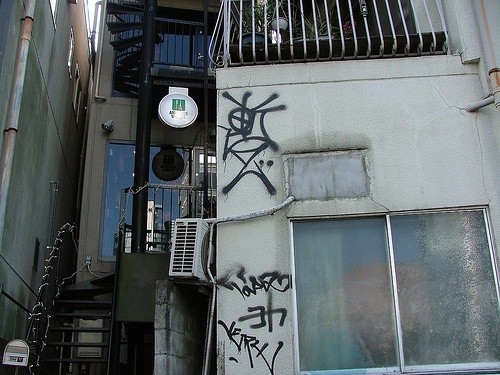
[343,18,359,37]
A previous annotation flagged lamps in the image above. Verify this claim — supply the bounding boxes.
[101,118,116,133]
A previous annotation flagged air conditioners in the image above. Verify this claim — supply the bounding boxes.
[170,217,216,282]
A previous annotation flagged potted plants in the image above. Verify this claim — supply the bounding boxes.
[238,2,275,44]
[288,8,340,42]
[267,0,291,31]
